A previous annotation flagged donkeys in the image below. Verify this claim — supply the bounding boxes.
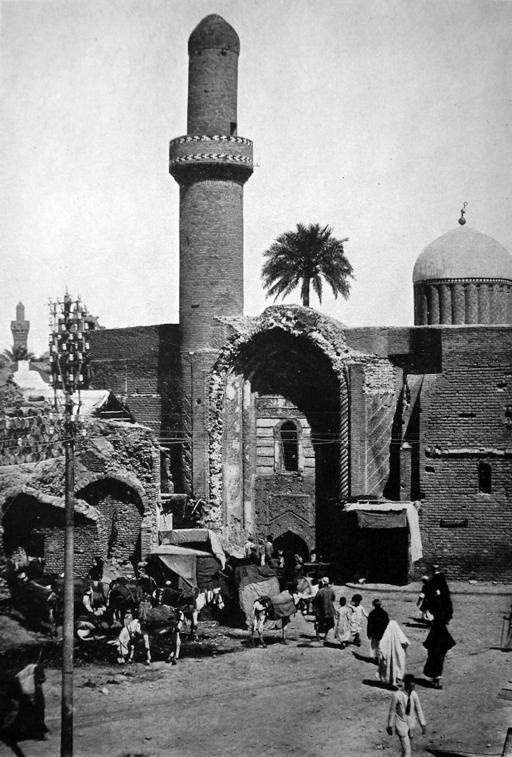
[252,589,307,648]
[17,568,157,640]
[152,586,225,641]
[107,604,185,666]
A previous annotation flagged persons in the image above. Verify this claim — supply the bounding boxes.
[312,576,337,641]
[422,616,458,689]
[240,532,311,578]
[427,565,454,625]
[376,620,410,687]
[333,595,353,650]
[415,575,430,621]
[366,599,391,665]
[347,593,369,648]
[387,674,427,757]
